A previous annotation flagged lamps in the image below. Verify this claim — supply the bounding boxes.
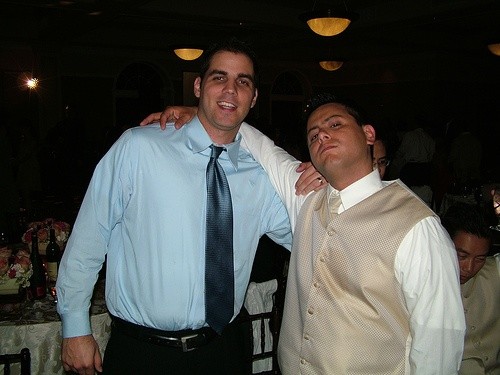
[174,47,204,61]
[319,58,344,71]
[299,0,359,37]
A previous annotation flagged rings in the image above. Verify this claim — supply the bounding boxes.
[316,178,324,185]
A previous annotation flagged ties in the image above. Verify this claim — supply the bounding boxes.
[328,194,342,221]
[205,145,234,329]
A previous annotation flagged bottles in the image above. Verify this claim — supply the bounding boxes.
[46,229,63,285]
[29,233,47,299]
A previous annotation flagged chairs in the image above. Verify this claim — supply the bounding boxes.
[250,303,281,375]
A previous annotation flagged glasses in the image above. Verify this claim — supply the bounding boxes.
[371,160,390,167]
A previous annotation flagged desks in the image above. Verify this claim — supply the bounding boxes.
[0,217,112,375]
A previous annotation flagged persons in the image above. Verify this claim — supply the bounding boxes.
[371,137,388,180]
[56,36,327,375]
[441,201,500,375]
[412,158,457,211]
[140,90,467,374]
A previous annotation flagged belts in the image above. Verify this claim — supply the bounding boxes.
[113,323,225,352]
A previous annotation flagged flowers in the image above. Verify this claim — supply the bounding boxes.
[0,246,33,288]
[22,218,70,255]
